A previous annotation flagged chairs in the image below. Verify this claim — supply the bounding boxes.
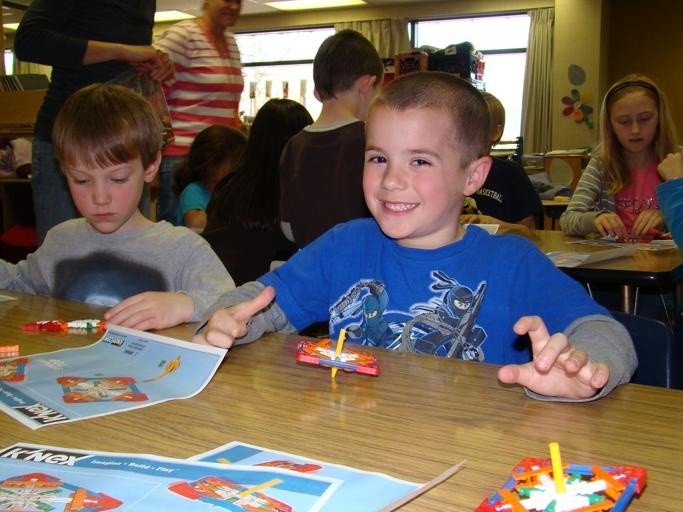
[610,310,673,389]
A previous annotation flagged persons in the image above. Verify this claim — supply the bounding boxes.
[14,1,175,246]
[559,76,683,327]
[148,0,248,226]
[172,30,386,340]
[459,91,545,237]
[181,69,639,405]
[0,80,236,335]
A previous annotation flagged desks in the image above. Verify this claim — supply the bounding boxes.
[482,228,683,335]
[0,287,683,512]
[539,199,571,231]
